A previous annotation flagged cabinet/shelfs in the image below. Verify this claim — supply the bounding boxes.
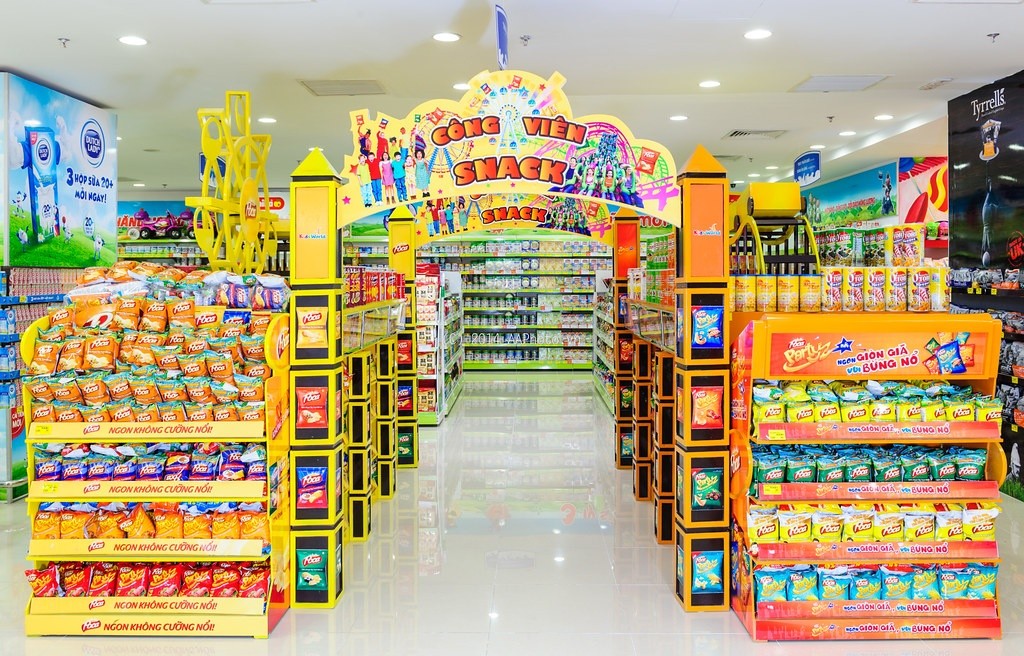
[0,226,1024,641]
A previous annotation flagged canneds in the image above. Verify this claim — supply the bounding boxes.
[627,232,675,309]
[430,245,486,271]
[729,222,951,313]
[463,296,539,361]
